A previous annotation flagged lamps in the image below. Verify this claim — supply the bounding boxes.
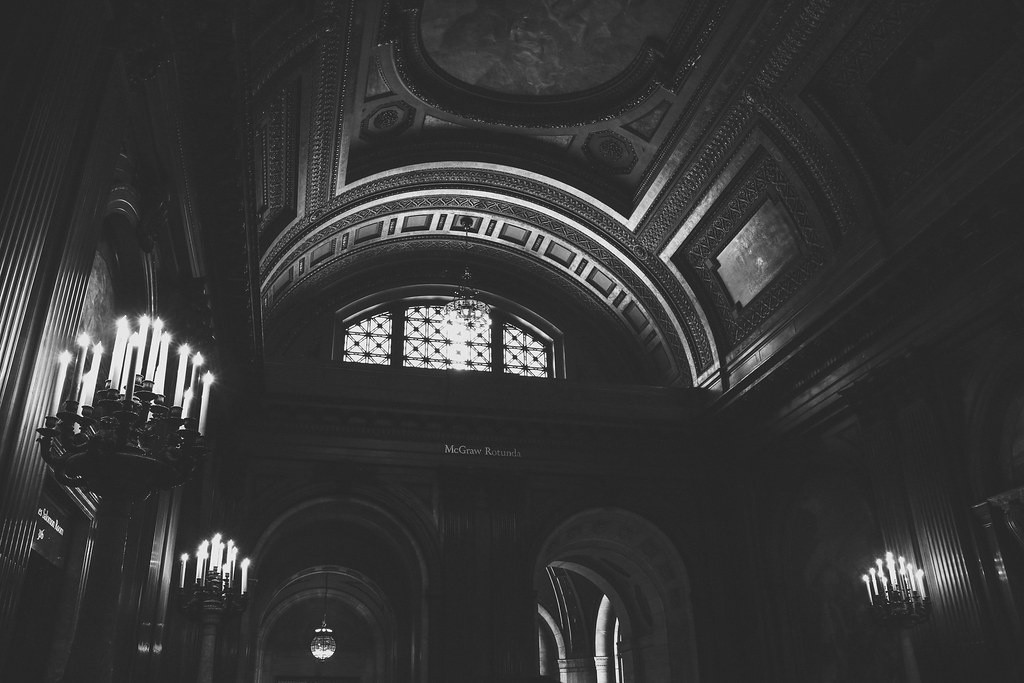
[442,216,492,342]
[309,573,336,665]
[860,549,930,683]
[34,313,212,683]
[169,532,250,683]
